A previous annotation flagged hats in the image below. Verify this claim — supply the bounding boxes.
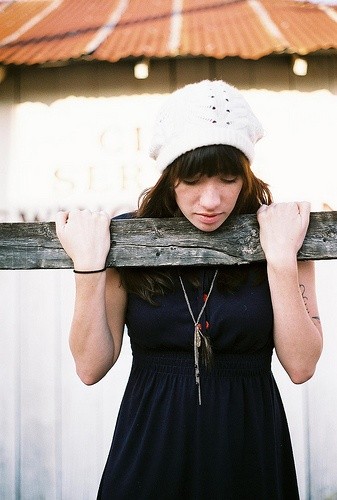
[145,78,265,174]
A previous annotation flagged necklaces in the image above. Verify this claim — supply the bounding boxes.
[179,266,220,405]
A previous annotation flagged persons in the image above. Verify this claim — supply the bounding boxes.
[56,77,323,500]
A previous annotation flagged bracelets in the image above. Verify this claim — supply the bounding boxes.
[73,267,107,274]
